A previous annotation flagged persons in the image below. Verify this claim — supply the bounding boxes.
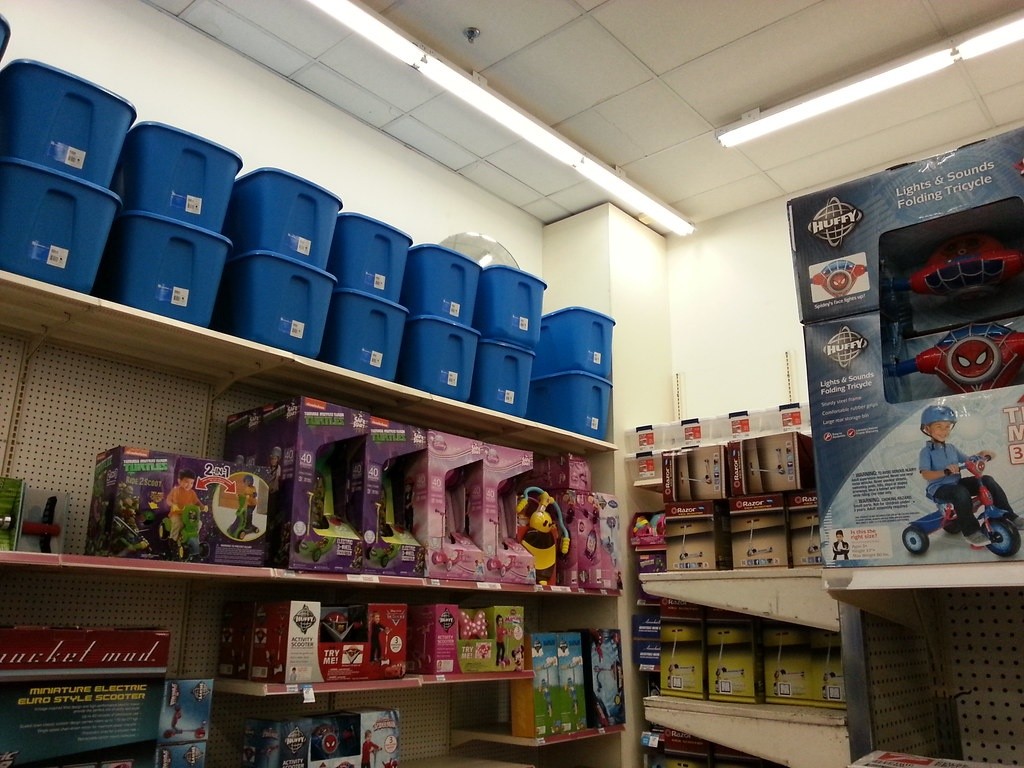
[833,530,849,560]
[243,475,258,529]
[496,615,507,666]
[290,668,297,683]
[370,613,389,663]
[361,730,381,768]
[919,405,1024,546]
[166,468,208,557]
[266,446,282,491]
[403,477,415,531]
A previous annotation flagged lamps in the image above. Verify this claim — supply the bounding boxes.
[713,12,1024,147]
[308,1,696,240]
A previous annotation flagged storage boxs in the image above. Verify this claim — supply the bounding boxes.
[1,12,1024,768]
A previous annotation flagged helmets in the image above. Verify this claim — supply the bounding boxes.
[921,404,957,426]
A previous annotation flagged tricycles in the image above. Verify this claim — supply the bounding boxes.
[902,456,1020,558]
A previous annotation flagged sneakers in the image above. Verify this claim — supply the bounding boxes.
[1009,515,1024,530]
[965,531,991,546]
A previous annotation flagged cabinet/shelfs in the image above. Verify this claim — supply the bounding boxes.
[631,478,777,767]
[0,269,626,768]
[637,559,1024,768]
